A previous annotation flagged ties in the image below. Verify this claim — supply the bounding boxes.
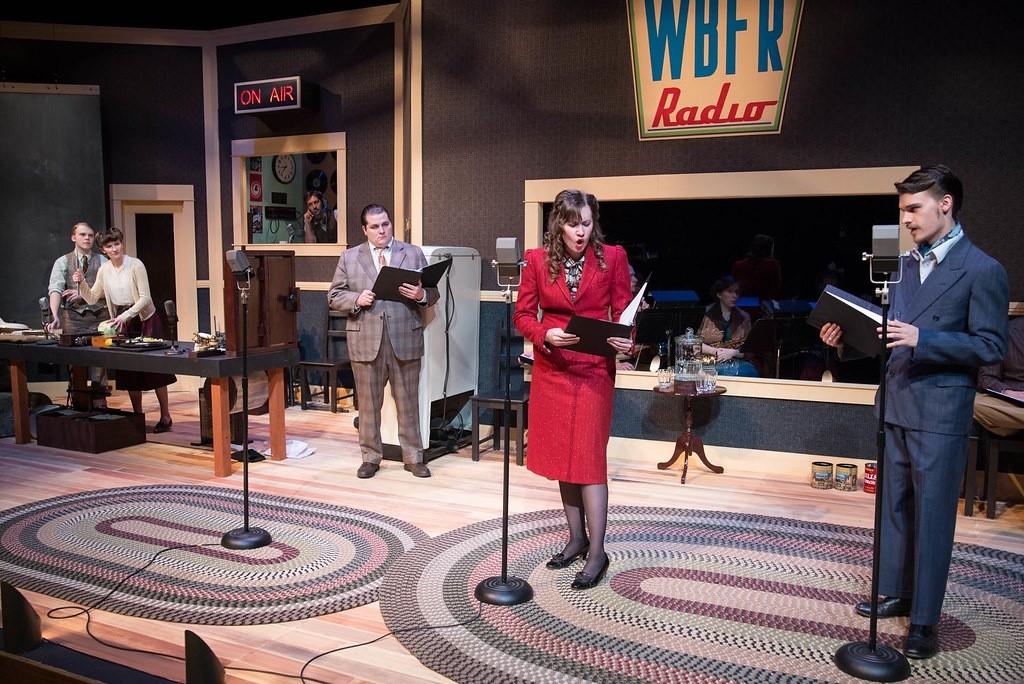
[378,249,386,269]
[82,255,88,275]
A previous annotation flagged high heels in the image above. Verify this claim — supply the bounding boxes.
[546,541,590,568]
[571,553,609,589]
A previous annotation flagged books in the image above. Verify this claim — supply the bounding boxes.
[371,259,451,303]
[557,282,647,359]
[987,388,1024,408]
[807,284,891,360]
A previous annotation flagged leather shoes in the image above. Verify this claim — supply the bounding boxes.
[153,417,172,432]
[404,464,431,477]
[357,462,379,478]
[855,597,911,618]
[904,623,936,659]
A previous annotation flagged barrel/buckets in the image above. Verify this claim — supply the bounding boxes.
[810,461,834,490]
[833,463,859,491]
[863,463,877,493]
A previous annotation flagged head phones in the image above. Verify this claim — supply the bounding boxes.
[321,193,328,208]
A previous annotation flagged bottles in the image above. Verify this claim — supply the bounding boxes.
[674,327,703,381]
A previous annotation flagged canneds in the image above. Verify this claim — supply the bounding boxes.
[863,463,877,493]
[811,462,858,491]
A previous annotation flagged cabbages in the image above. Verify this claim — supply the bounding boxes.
[98,320,120,335]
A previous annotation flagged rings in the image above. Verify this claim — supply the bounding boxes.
[829,338,832,341]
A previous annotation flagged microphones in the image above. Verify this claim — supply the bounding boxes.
[496,237,521,276]
[164,300,179,324]
[314,206,327,215]
[872,224,900,273]
[39,297,53,317]
[226,249,255,281]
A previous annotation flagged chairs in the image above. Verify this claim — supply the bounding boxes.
[468,320,529,466]
[299,304,359,413]
[982,302,1024,519]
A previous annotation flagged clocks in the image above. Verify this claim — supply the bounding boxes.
[272,154,296,184]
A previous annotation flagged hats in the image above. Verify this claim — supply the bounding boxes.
[710,276,733,294]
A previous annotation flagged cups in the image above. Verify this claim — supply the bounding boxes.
[657,365,675,392]
[694,365,717,394]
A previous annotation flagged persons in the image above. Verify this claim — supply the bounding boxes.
[44,223,178,434]
[513,189,637,589]
[327,204,441,479]
[696,275,761,378]
[303,191,338,244]
[819,164,1024,657]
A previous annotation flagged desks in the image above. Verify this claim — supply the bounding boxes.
[0,333,299,478]
[653,383,727,484]
